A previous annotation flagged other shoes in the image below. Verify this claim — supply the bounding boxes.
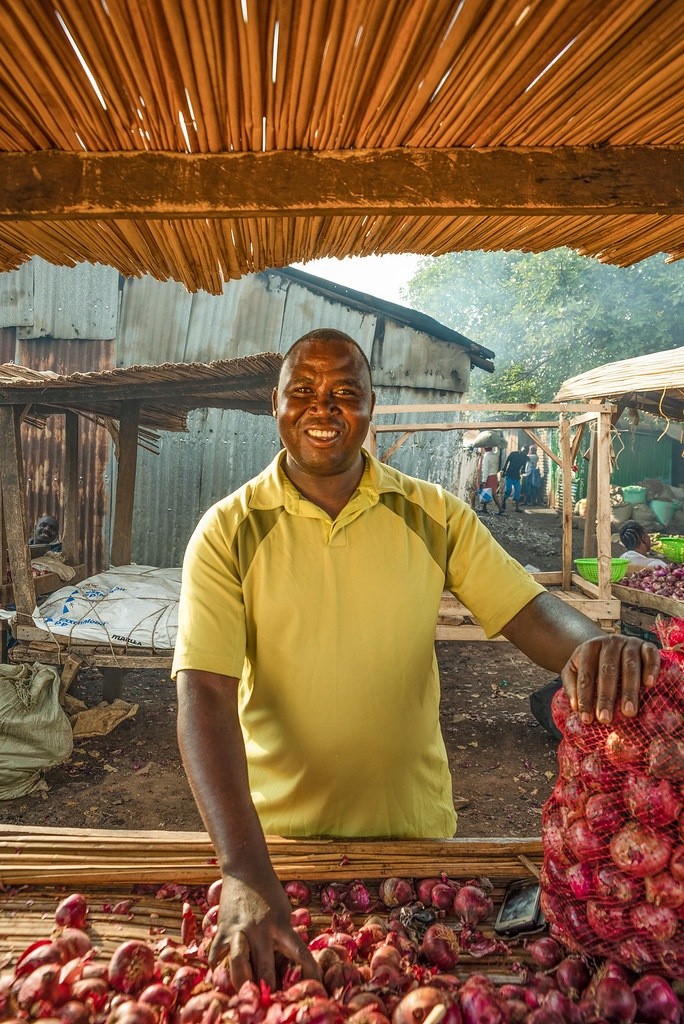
[528,502,535,506]
[500,511,508,516]
[518,502,525,506]
[515,508,522,512]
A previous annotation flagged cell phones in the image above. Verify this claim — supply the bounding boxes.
[495,877,544,937]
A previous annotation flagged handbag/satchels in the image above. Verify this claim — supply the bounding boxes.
[476,487,493,504]
[532,462,542,487]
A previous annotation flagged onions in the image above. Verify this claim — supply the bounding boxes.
[0,876,683,1024]
[615,562,684,605]
[537,645,684,980]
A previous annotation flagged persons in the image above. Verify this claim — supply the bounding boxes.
[25,517,64,548]
[616,524,667,572]
[172,328,659,990]
[479,444,542,512]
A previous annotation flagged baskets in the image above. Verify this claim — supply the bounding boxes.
[573,557,631,585]
[659,537,684,563]
